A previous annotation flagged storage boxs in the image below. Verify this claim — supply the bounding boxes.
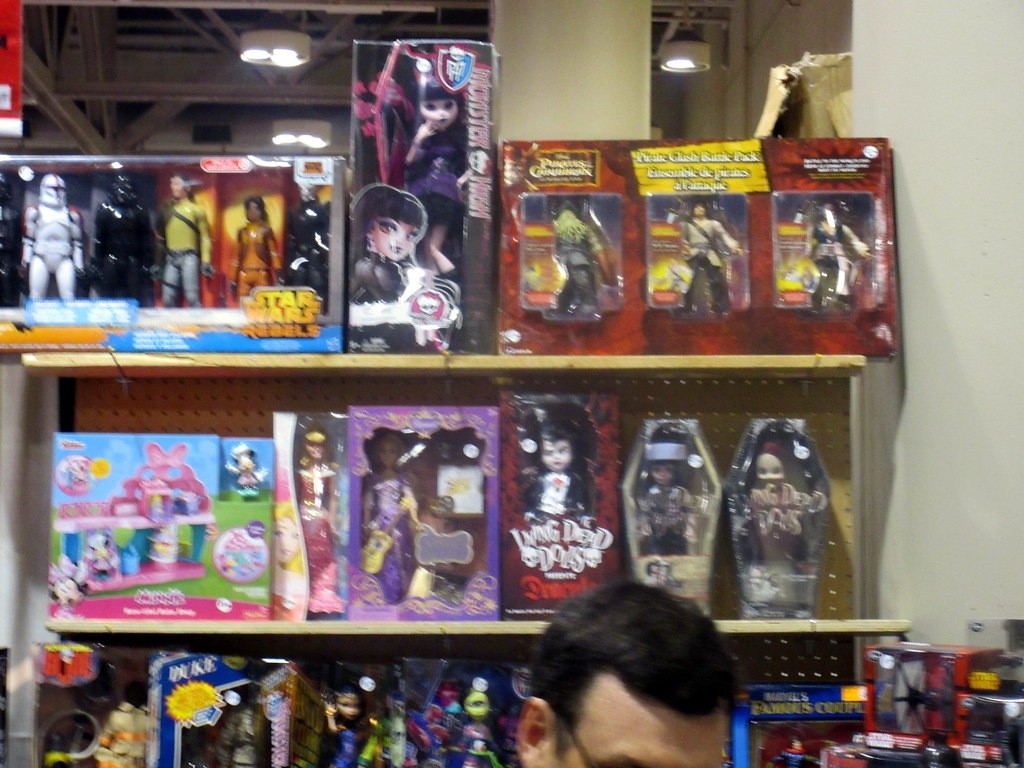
[0,37,1004,767]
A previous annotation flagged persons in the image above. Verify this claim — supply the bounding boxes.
[156,176,215,307]
[282,186,327,304]
[808,203,871,311]
[90,178,157,304]
[517,581,735,768]
[0,173,85,309]
[550,199,614,319]
[230,196,282,308]
[402,79,468,278]
[95,416,1024,768]
[679,203,743,316]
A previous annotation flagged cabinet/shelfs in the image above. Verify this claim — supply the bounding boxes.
[17,345,915,649]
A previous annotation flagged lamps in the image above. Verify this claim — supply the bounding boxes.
[236,10,313,68]
[659,21,712,73]
